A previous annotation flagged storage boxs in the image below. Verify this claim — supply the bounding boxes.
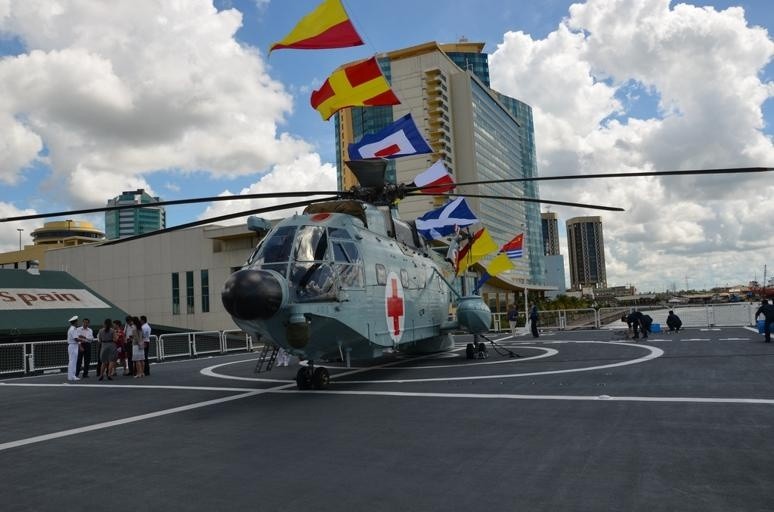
[650,323,660,333]
[756,319,774,333]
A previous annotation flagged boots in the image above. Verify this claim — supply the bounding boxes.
[632,332,648,338]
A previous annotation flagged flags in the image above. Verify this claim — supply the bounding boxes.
[311,55,401,122]
[348,114,434,160]
[414,196,480,242]
[454,227,498,277]
[473,252,518,292]
[268,0,364,55]
[498,233,525,259]
[391,159,458,204]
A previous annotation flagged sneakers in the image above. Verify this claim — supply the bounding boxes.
[123,371,150,378]
[96,373,117,380]
[277,362,289,366]
[72,375,89,380]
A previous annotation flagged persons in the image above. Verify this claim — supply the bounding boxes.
[666,311,682,331]
[755,300,774,343]
[76,318,93,377]
[507,304,518,338]
[67,316,88,381]
[622,313,648,338]
[96,315,152,382]
[276,347,289,367]
[528,299,540,337]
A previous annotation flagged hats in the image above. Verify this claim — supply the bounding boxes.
[69,316,78,323]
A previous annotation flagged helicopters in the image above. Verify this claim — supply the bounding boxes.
[2,156,774,390]
